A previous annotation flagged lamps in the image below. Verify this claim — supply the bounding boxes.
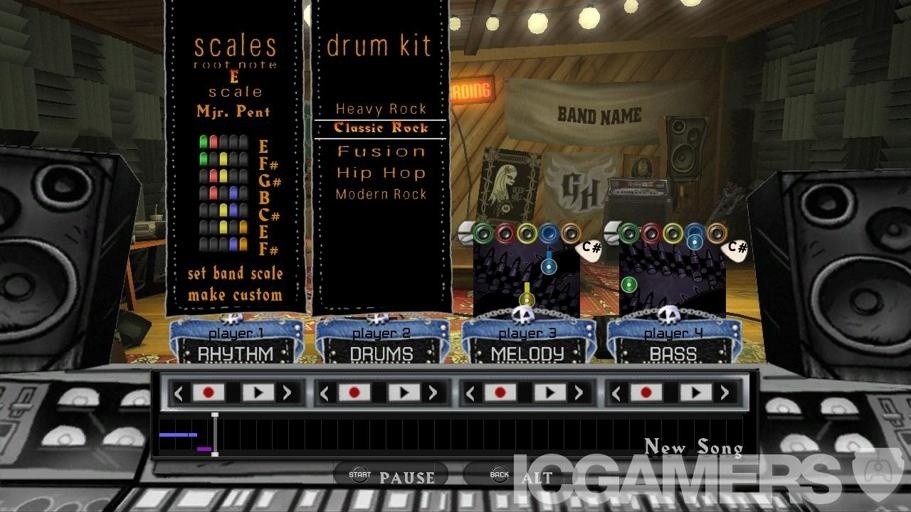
[449,0,705,40]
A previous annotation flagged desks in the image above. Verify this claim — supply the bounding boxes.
[122,239,167,311]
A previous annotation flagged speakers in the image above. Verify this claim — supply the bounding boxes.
[665,115,711,183]
[747,165,911,388]
[607,177,672,221]
[0,144,143,374]
